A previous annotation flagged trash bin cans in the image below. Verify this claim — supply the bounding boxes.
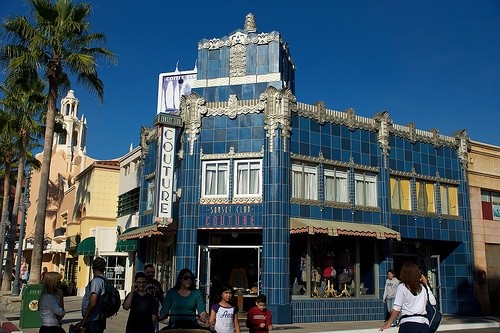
[19,284,44,328]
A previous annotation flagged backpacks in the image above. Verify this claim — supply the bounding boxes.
[89,275,121,318]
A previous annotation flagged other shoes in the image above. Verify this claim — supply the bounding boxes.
[391,317,400,327]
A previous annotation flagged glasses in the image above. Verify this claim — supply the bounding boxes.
[182,276,195,281]
[134,281,148,285]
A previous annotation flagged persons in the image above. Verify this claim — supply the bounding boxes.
[74,257,107,333]
[144,264,165,308]
[228,267,248,288]
[39,272,67,333]
[382,269,402,327]
[9,257,50,287]
[378,260,436,333]
[245,294,273,333]
[200,285,241,333]
[121,272,159,333]
[301,256,355,297]
[152,268,207,327]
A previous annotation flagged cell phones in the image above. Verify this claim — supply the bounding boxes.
[135,282,138,289]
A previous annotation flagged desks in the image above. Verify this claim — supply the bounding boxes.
[234,290,259,313]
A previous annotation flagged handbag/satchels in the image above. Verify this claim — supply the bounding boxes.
[69,324,86,333]
[426,301,442,333]
